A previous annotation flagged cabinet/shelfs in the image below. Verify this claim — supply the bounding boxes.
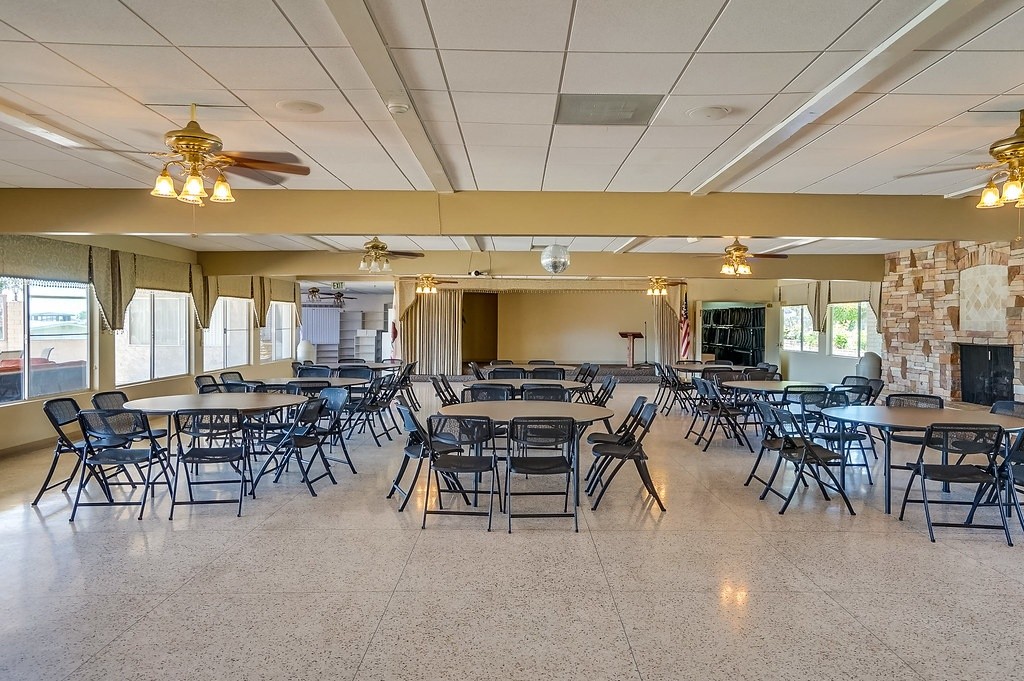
[301,303,394,378]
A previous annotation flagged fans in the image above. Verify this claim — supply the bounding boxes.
[321,236,425,261]
[695,237,788,259]
[318,292,357,300]
[943,109,1024,200]
[62,103,311,175]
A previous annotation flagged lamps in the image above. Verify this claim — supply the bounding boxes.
[358,253,392,273]
[720,255,753,275]
[413,274,438,295]
[149,161,236,205]
[646,275,669,297]
[306,287,322,304]
[975,165,1024,208]
[332,300,346,312]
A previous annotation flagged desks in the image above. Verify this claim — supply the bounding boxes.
[438,400,615,505]
[721,381,853,449]
[312,363,402,376]
[123,392,308,497]
[463,378,586,395]
[821,405,1024,514]
[483,364,577,378]
[670,365,759,408]
[249,377,370,445]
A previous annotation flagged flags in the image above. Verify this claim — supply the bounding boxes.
[679,292,690,365]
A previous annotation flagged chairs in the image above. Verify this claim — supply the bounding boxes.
[654,360,1024,548]
[32,360,422,520]
[386,360,666,532]
